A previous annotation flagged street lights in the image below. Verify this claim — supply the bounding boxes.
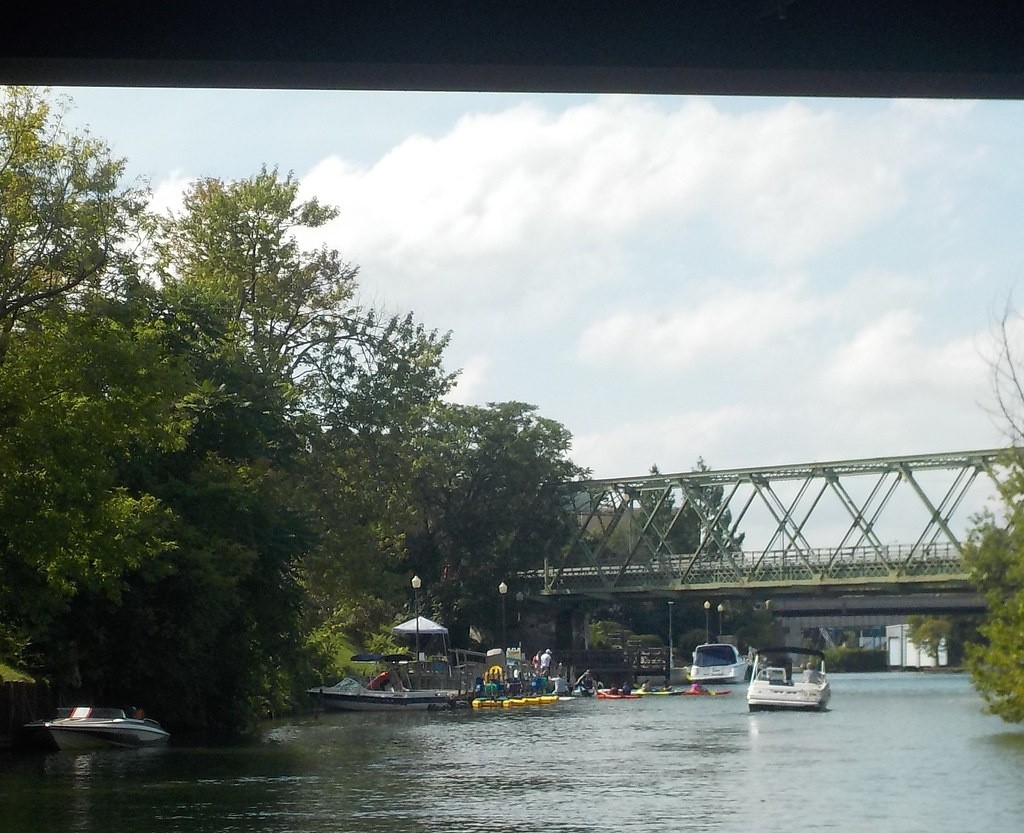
[704,600,711,643]
[412,575,421,661]
[717,604,723,643]
[499,582,507,651]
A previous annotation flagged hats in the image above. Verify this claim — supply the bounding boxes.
[546,649,552,653]
[584,670,592,676]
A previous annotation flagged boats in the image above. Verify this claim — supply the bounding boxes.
[747,647,831,713]
[690,642,748,684]
[306,653,465,713]
[468,648,560,707]
[595,692,639,699]
[22,707,170,751]
[571,690,595,697]
[683,690,732,695]
[632,689,685,695]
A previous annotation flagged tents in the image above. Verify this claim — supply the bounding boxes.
[391,617,453,678]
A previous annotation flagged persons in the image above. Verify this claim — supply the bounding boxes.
[623,682,631,694]
[691,681,703,691]
[532,649,552,671]
[580,670,593,689]
[548,674,566,695]
[803,662,817,684]
[608,684,618,694]
[641,680,649,691]
